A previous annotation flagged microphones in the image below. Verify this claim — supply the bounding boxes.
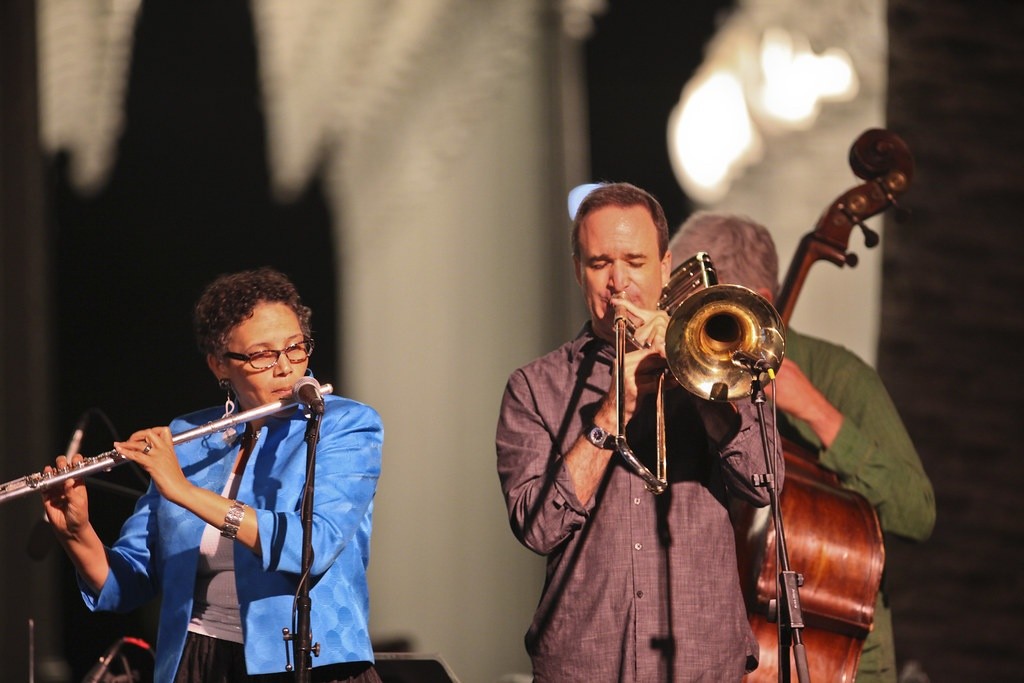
[732,350,774,376]
[44,411,90,522]
[84,640,123,683]
[292,377,324,416]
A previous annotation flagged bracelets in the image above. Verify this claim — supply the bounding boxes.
[219,500,248,542]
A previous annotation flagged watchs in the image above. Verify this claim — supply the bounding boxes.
[582,420,617,452]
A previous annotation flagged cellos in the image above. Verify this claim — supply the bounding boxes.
[738,128,908,683]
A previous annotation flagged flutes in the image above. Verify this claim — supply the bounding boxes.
[0,384,333,503]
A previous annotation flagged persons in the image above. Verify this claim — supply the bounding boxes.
[495,182,935,683]
[40,268,384,683]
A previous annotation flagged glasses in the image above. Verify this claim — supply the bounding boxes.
[225,334,315,369]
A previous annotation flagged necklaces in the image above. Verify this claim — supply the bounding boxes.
[242,430,260,443]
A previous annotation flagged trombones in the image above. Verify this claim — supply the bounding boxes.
[612,251,786,495]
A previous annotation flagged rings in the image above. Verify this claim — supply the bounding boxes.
[142,444,152,455]
